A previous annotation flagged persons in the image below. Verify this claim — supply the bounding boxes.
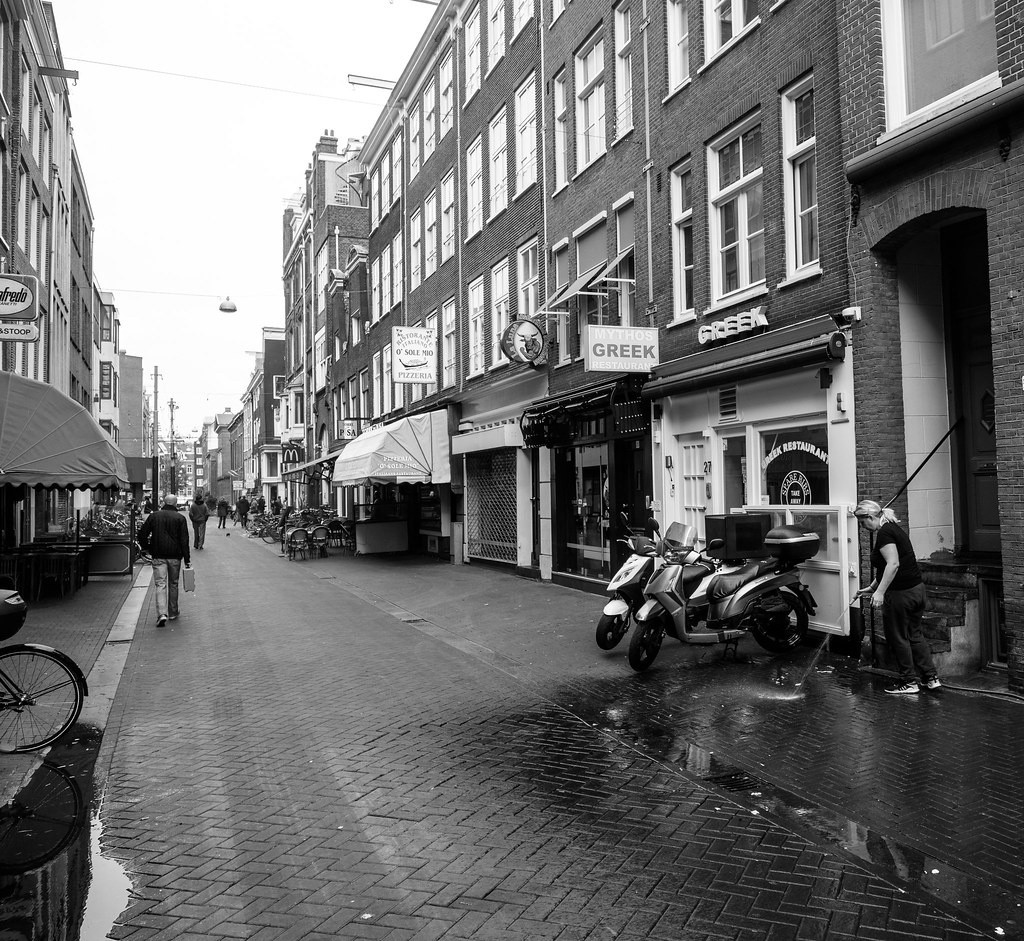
[270,496,288,516]
[251,496,265,513]
[217,498,228,528]
[138,494,191,627]
[189,494,211,550]
[370,490,381,519]
[233,496,250,528]
[391,490,397,503]
[856,500,941,693]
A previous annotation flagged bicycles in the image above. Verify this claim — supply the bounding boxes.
[0,589,89,756]
[0,756,85,882]
[259,497,331,544]
[67,499,154,565]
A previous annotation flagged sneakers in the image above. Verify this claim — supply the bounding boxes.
[884,679,919,693]
[919,675,941,690]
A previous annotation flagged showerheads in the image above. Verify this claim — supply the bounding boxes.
[849,589,872,606]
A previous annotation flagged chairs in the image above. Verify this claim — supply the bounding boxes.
[0,555,22,591]
[29,551,66,601]
[285,518,353,560]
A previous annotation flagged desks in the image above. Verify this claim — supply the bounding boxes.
[6,545,93,598]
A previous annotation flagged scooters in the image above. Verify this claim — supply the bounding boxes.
[595,509,822,673]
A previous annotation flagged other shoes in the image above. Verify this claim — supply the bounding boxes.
[200,547,204,550]
[155,614,167,627]
[194,545,198,549]
[169,610,180,620]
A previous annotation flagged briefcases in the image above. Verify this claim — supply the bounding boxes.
[183,563,195,592]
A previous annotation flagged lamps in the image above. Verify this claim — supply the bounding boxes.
[654,404,662,419]
[220,297,237,312]
[819,367,833,389]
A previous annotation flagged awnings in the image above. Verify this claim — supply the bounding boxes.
[0,370,130,492]
[333,409,451,485]
